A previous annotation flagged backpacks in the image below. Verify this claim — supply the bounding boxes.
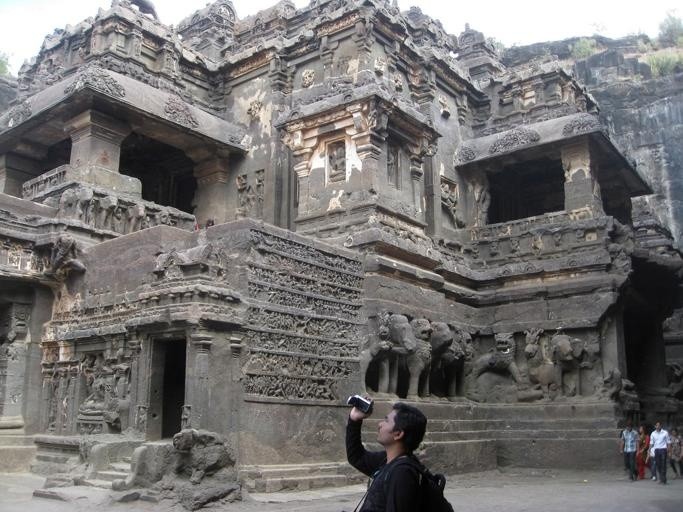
[385,462,455,511]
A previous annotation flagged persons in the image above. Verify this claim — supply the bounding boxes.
[617,420,682,485]
[344,393,455,511]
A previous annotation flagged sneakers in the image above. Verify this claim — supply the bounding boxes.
[622,470,682,485]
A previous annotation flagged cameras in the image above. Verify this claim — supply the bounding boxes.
[347,395,372,414]
